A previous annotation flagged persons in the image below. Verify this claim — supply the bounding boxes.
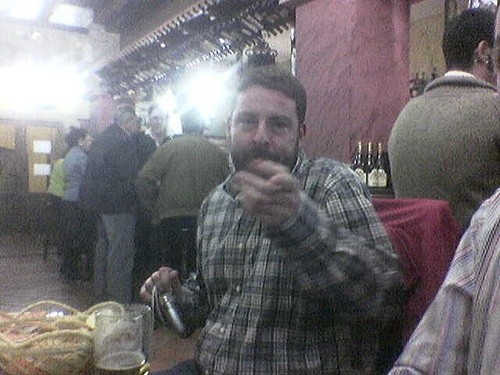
[61,126,98,279]
[47,151,65,257]
[389,0,499,375]
[139,63,404,374]
[135,104,230,313]
[78,106,140,307]
[385,6,499,243]
[131,96,172,165]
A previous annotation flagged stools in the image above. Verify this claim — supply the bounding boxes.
[43,199,61,263]
[158,225,200,284]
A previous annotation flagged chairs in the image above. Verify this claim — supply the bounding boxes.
[366,199,460,372]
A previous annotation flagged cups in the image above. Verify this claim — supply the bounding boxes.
[95,307,154,375]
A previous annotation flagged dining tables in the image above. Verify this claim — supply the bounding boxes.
[1,310,150,375]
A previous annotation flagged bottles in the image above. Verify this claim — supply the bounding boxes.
[159,291,192,341]
[363,142,379,194]
[96,1,291,106]
[352,142,366,188]
[408,67,439,97]
[374,143,391,194]
